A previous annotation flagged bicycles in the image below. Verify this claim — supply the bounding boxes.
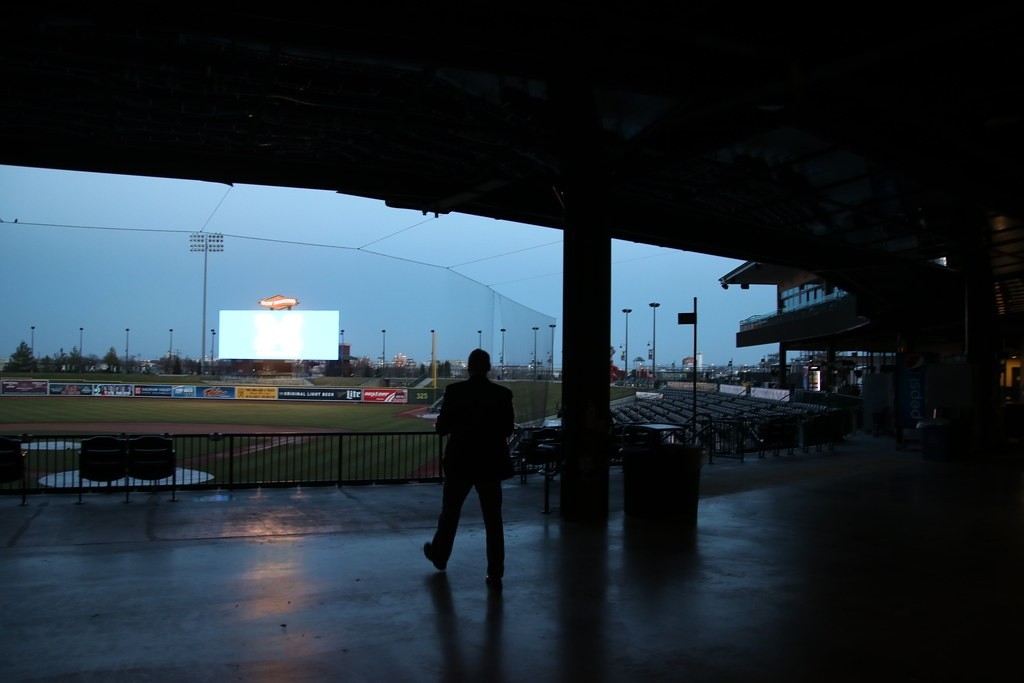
[515,419,564,515]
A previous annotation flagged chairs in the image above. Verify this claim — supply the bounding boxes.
[79,436,130,504]
[0,437,30,505]
[518,415,843,486]
[127,437,178,503]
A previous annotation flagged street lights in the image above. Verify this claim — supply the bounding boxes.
[340,329,345,377]
[532,326,539,380]
[549,324,556,376]
[382,330,386,367]
[168,328,173,374]
[430,329,435,404]
[31,326,35,360]
[500,328,506,380]
[79,327,84,374]
[622,308,632,386]
[649,302,660,379]
[125,328,130,373]
[210,329,217,374]
[478,330,482,349]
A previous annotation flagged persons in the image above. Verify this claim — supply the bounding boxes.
[424,348,515,589]
[61,385,80,395]
[101,385,131,395]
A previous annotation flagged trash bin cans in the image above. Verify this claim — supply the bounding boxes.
[620,422,701,543]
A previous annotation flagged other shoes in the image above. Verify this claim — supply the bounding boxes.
[485,576,503,592]
[423,542,448,573]
